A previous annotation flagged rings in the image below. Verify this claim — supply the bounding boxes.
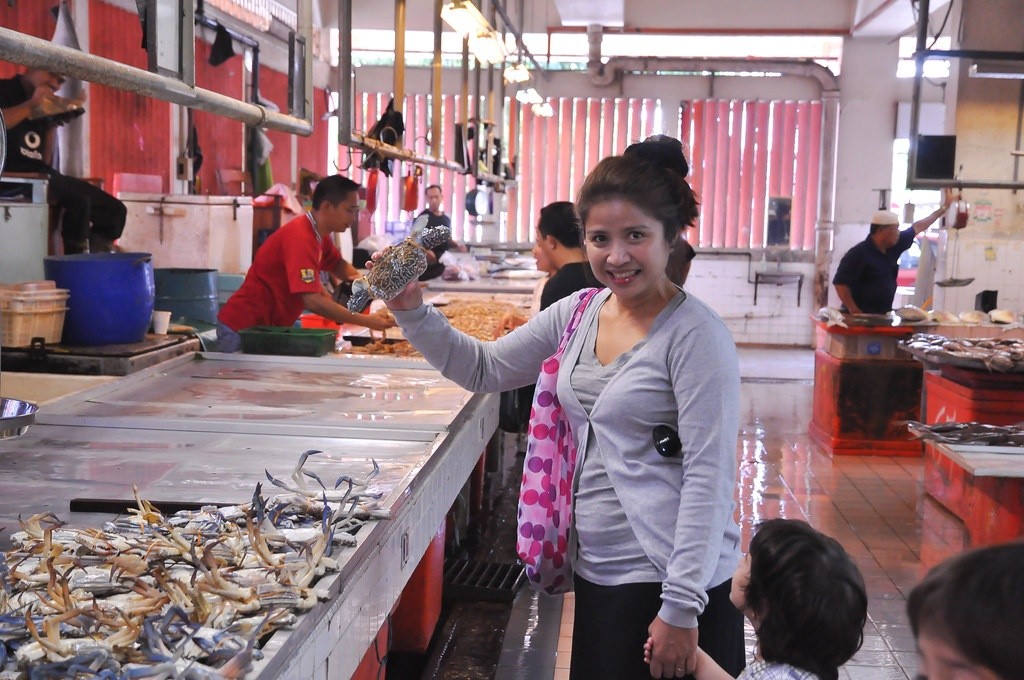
[676,668,686,672]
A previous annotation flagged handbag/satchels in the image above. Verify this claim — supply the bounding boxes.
[498,388,521,434]
[515,287,605,595]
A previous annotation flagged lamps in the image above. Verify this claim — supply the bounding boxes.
[440,0,556,118]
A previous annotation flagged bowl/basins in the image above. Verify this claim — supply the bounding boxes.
[300,313,341,337]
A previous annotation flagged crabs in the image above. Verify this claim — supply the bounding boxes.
[0,450,396,680]
[346,224,451,313]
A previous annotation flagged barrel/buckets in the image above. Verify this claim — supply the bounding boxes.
[153,268,220,326]
[44,252,156,346]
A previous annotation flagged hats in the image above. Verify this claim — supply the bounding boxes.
[871,210,899,225]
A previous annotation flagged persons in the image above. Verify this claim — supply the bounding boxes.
[365,135,746,680]
[645,519,868,680]
[906,542,1024,680]
[409,185,468,285]
[833,187,957,316]
[217,174,393,353]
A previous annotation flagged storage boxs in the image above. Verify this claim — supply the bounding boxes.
[301,305,371,336]
[0,287,71,348]
[239,324,339,358]
[118,192,253,275]
[942,366,1023,389]
[349,518,446,680]
